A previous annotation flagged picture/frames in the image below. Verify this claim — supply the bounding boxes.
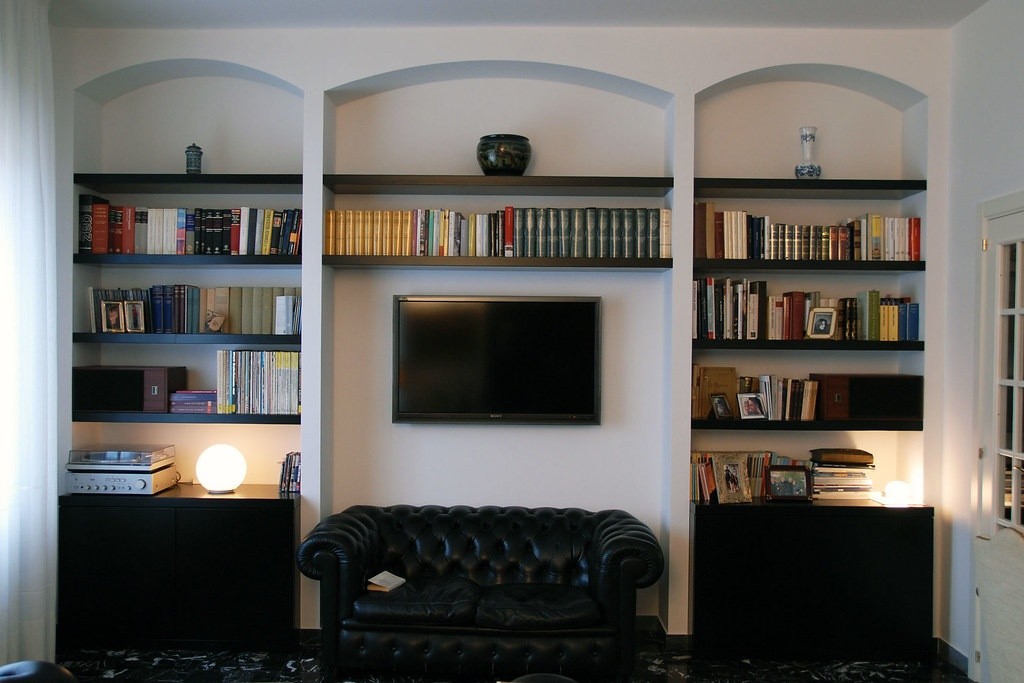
[736,392,769,420]
[709,453,753,504]
[708,392,735,419]
[804,307,840,340]
[101,299,126,332]
[765,463,813,503]
[124,299,146,333]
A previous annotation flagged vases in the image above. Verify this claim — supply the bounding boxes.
[476,134,533,177]
[792,125,822,179]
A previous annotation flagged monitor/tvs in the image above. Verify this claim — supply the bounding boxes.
[391,293,603,426]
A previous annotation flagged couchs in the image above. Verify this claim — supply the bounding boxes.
[293,503,665,683]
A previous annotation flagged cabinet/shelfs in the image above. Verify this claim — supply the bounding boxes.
[685,496,934,679]
[54,482,300,671]
[70,170,304,425]
[688,177,936,432]
[322,172,674,273]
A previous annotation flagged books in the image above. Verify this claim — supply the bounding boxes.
[691,202,920,504]
[366,570,406,592]
[324,207,672,258]
[77,193,303,493]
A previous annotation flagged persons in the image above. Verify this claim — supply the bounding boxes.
[748,398,764,415]
[730,474,737,491]
[744,399,750,416]
[724,466,733,485]
[106,305,120,329]
[814,318,827,334]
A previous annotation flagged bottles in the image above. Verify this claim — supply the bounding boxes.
[476,133,532,176]
[793,126,822,178]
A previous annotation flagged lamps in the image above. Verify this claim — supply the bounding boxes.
[195,443,246,494]
